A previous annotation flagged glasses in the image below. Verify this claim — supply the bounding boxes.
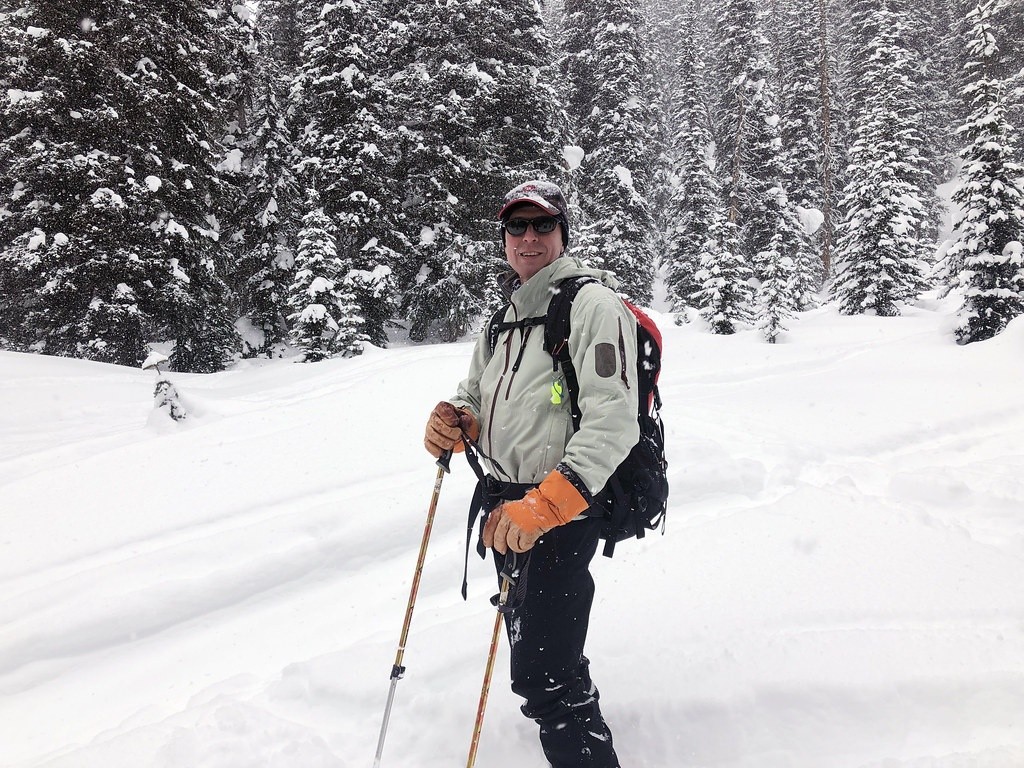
[501,214,561,236]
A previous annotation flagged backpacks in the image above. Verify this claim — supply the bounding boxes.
[489,276,669,558]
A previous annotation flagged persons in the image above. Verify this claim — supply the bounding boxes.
[424,181,641,767]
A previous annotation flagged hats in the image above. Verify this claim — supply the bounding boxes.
[497,180,569,234]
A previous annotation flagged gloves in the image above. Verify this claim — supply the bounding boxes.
[424,401,480,458]
[482,468,590,555]
